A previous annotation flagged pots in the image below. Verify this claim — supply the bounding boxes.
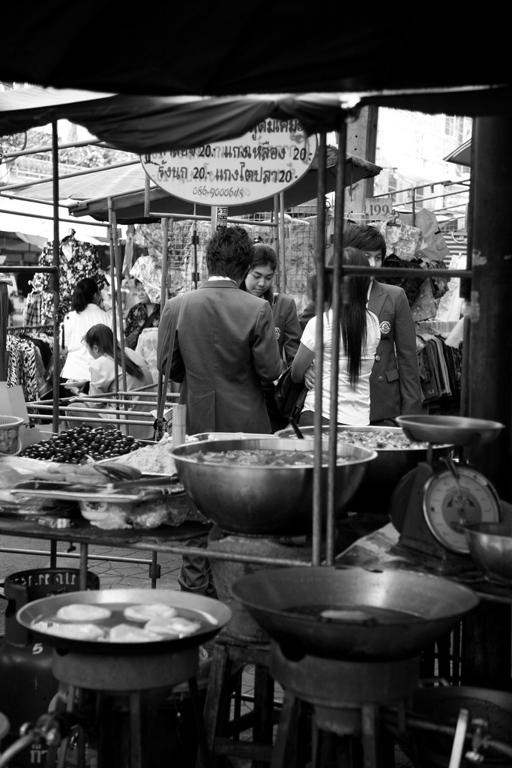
[233,563,475,662]
[18,585,234,657]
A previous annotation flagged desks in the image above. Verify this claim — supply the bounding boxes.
[1,514,511,602]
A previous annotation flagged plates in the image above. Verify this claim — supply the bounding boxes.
[60,380,88,388]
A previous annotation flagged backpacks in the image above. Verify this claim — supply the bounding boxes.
[268,361,309,430]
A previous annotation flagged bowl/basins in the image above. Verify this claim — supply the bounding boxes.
[167,435,377,538]
[457,521,511,587]
[277,425,453,510]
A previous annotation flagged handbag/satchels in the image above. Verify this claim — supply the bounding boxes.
[155,410,172,441]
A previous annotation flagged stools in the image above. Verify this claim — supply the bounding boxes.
[195,632,303,768]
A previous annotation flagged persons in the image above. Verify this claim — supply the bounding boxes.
[288,226,424,569]
[63,280,158,444]
[155,224,308,600]
